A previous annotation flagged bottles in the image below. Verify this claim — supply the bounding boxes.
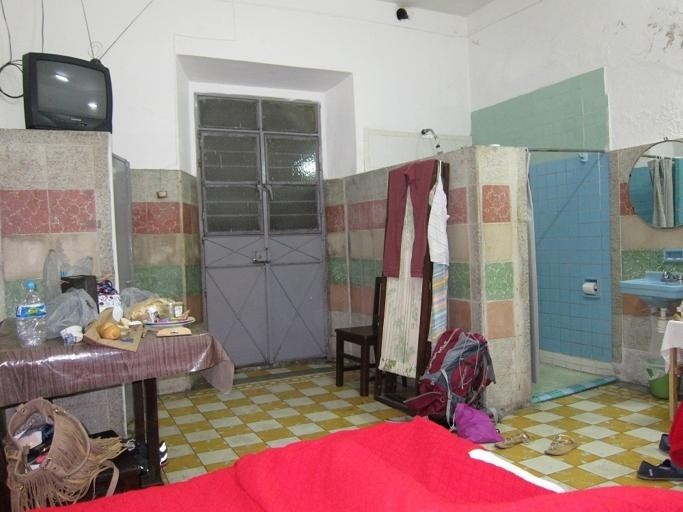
[15,280,48,349]
[42,248,65,289]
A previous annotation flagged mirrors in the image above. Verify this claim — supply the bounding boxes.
[627,138,683,229]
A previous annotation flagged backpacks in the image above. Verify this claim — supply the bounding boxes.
[400,327,496,423]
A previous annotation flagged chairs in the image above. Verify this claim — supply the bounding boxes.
[334,276,405,398]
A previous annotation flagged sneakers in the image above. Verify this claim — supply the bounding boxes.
[160,441,167,466]
[661,434,671,449]
[638,458,683,481]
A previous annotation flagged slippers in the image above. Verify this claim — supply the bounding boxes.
[544,433,579,455]
[495,434,535,449]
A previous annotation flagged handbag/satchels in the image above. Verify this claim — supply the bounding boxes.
[4,395,121,512]
[97,281,123,320]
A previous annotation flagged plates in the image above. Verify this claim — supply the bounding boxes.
[143,316,196,329]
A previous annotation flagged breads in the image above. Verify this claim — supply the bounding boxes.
[100,321,120,339]
[125,296,163,320]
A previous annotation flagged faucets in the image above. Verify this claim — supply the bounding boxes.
[661,270,676,281]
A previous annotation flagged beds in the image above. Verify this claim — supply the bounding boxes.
[17,412,683,511]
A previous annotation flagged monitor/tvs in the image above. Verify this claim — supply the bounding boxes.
[22,51,114,131]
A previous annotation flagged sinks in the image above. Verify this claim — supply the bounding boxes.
[619,278,683,302]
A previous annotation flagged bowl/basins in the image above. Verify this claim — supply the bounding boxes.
[157,301,185,318]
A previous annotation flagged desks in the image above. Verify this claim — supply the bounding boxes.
[0,315,213,489]
